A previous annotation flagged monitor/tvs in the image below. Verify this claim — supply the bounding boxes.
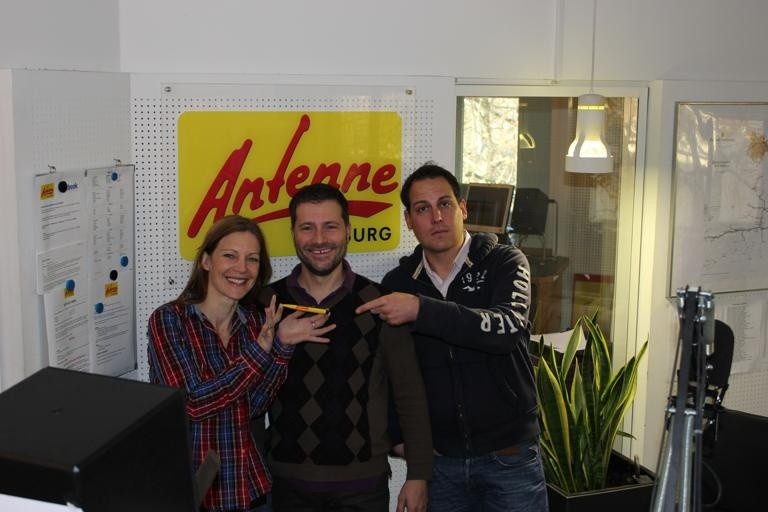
[464,182,515,234]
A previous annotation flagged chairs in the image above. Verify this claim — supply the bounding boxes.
[571,273,615,340]
[666,320,734,443]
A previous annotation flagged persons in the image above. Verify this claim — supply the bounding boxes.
[352,159,552,512]
[246,179,435,511]
[139,212,337,511]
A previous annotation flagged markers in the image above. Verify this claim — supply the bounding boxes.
[281,304,328,317]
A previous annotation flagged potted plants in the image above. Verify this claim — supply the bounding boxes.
[532,310,662,512]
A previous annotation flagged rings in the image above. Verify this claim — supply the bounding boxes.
[311,322,316,328]
[266,325,274,329]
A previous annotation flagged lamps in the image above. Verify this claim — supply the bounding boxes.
[564,0,615,174]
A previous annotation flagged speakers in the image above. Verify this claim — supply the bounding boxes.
[511,188,548,235]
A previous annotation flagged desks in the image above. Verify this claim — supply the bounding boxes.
[524,255,569,335]
